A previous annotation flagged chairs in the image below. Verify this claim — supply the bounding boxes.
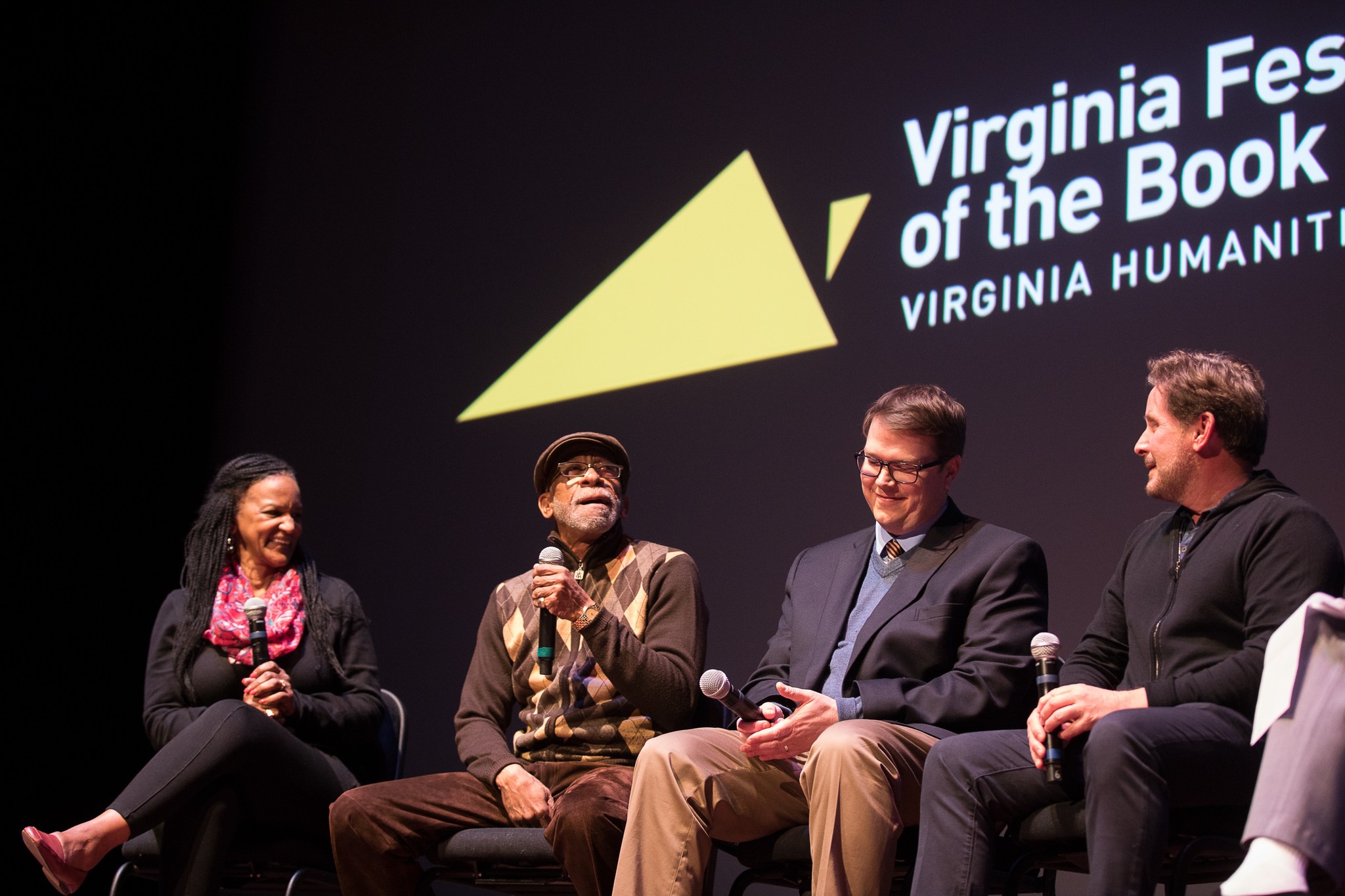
[720,655,1259,896]
[110,688,408,896]
[427,699,719,896]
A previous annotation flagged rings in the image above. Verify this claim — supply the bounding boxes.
[280,680,286,690]
[784,745,789,752]
[538,597,545,608]
[266,709,275,718]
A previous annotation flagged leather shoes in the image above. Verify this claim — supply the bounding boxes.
[21,826,88,896]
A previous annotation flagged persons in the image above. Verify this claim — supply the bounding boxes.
[22,453,396,896]
[1209,612,1345,896]
[329,432,708,896]
[610,384,1047,896]
[923,349,1345,896]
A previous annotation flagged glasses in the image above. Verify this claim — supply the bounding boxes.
[547,461,624,493]
[855,447,948,483]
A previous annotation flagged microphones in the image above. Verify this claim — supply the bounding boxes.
[244,597,271,698]
[538,546,564,677]
[1030,631,1061,782]
[699,669,776,724]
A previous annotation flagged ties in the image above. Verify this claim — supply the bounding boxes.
[885,539,900,563]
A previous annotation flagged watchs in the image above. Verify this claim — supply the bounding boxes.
[571,602,603,632]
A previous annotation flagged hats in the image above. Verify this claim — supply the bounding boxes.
[534,432,631,495]
